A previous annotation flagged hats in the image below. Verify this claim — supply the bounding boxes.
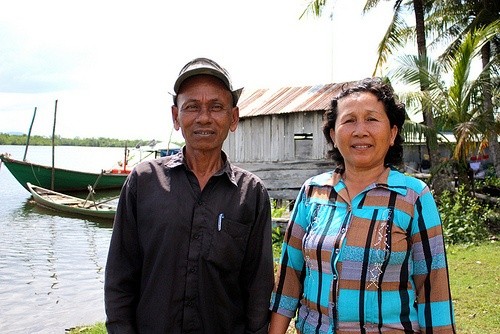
[174,57,232,96]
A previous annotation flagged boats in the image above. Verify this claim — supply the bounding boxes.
[0,152,129,195]
[26,171,117,219]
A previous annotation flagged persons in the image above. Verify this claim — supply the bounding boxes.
[465,162,475,195]
[268,76,455,334]
[104,55,276,334]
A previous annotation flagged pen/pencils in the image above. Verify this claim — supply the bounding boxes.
[217,214,226,231]
